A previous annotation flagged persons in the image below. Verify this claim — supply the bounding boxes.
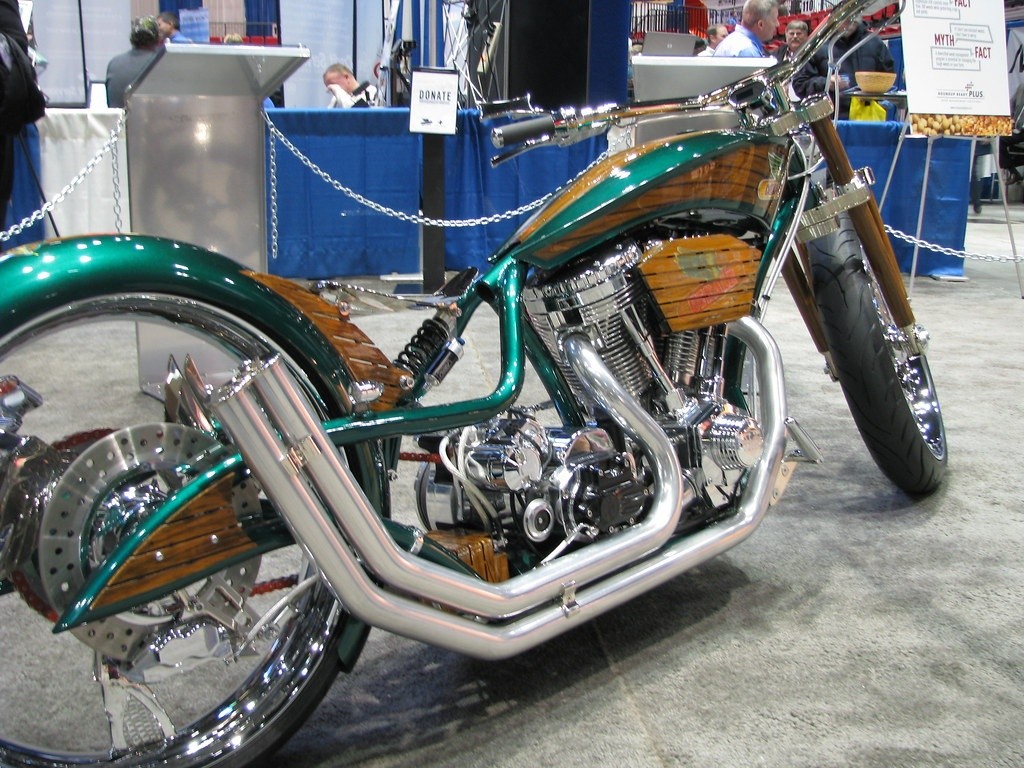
[697,0,895,120]
[224,33,243,44]
[0,0,46,254]
[105,13,190,108]
[324,64,385,108]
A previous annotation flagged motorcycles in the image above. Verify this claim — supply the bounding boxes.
[0,0,948,768]
